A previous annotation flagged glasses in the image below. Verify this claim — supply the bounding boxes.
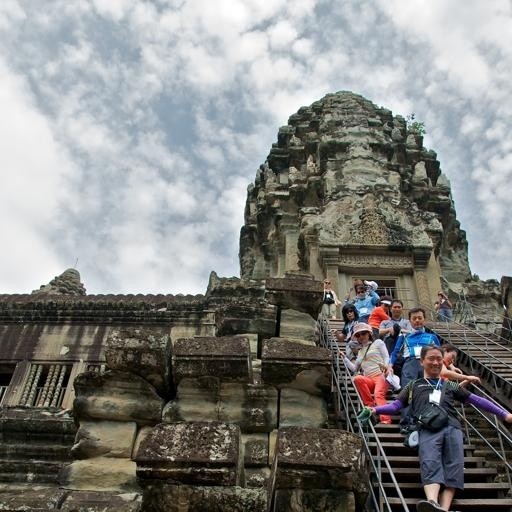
[354,330,367,338]
[346,310,353,314]
[358,291,364,294]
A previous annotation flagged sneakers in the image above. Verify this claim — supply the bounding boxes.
[415,499,447,512]
[399,422,412,434]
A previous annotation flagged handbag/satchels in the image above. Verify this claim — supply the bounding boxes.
[413,401,449,433]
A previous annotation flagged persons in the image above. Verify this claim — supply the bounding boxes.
[345,279,378,303]
[320,277,342,320]
[432,291,455,323]
[352,286,381,320]
[379,300,412,366]
[337,322,395,425]
[389,307,441,434]
[368,295,393,340]
[424,344,481,387]
[328,304,368,343]
[363,344,512,512]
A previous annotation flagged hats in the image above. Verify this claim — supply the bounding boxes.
[364,280,378,290]
[350,322,374,341]
[380,300,392,306]
[387,373,402,391]
